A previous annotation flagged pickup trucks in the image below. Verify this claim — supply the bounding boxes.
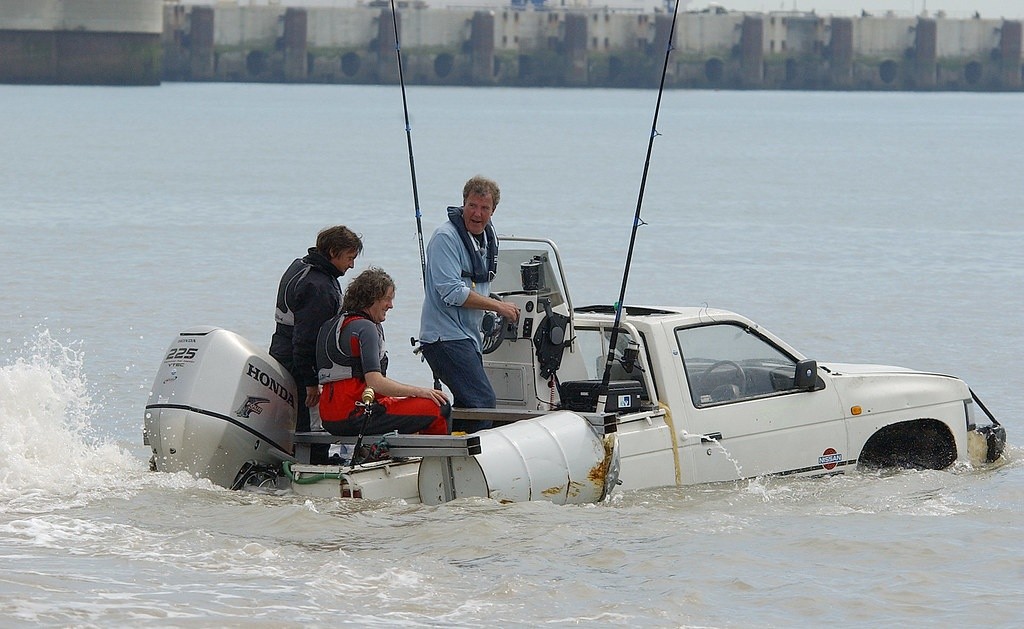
[144,234,1004,502]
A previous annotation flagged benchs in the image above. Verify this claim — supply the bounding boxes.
[452,408,548,429]
[294,431,387,462]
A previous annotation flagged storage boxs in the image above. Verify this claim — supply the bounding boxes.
[560,380,643,413]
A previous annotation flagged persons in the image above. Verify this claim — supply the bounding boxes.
[314,266,450,436]
[419,174,524,432]
[266,227,365,465]
